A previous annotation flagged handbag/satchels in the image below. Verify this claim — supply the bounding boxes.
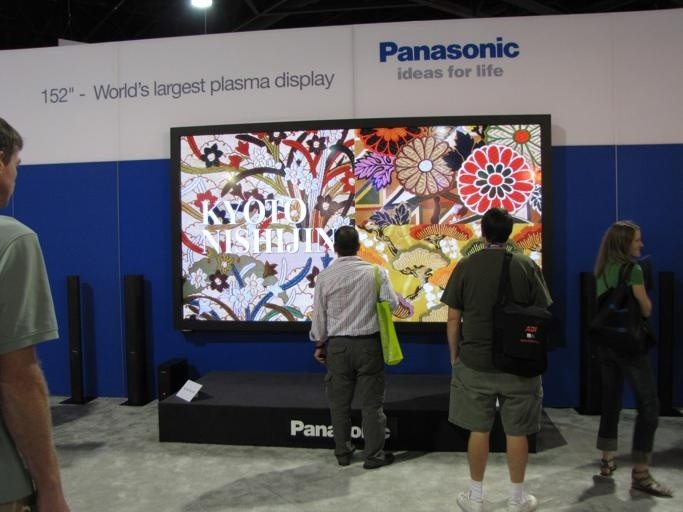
[490,296,549,382]
[374,298,407,369]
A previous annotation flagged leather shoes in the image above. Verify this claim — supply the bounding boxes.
[363,452,395,470]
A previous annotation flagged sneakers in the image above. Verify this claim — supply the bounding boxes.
[506,494,538,512]
[455,489,484,512]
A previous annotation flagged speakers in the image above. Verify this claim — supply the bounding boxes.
[578,271,604,412]
[66,275,84,402]
[123,274,152,403]
[657,270,674,415]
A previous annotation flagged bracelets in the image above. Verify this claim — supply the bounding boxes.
[315,345,323,351]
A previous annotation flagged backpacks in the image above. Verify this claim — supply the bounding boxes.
[594,258,655,361]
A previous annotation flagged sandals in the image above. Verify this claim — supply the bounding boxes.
[599,455,620,478]
[631,467,673,498]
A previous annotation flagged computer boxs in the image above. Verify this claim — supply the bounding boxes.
[157,357,187,401]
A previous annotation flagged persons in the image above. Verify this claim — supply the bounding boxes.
[308,226,396,470]
[438,208,553,511]
[0,119,70,512]
[594,220,673,498]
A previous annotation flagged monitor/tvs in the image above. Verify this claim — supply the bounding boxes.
[168,112,553,336]
